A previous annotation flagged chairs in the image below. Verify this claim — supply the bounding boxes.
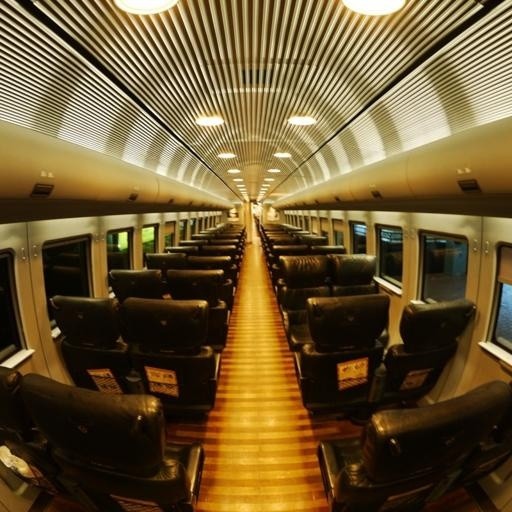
[109,222,244,343]
[2,295,222,512]
[257,223,391,348]
[294,295,512,511]
